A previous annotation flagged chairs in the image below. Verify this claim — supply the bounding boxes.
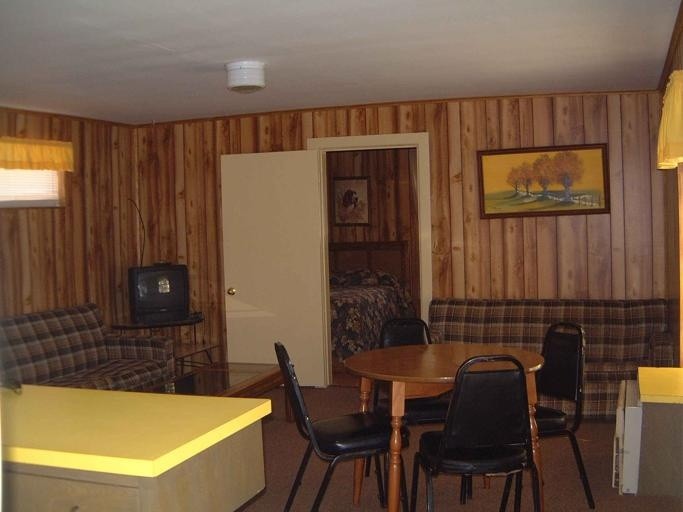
[274,342,410,511]
[372,318,431,410]
[499,322,595,510]
[410,355,542,510]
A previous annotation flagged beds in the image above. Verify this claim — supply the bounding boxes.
[328,239,412,376]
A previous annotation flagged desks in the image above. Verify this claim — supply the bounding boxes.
[1,382,272,512]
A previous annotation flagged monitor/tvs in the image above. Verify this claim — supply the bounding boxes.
[128,264,189,322]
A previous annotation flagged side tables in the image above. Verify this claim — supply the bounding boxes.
[111,312,213,379]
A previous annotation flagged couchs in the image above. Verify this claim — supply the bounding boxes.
[0,302,177,393]
[426,297,675,422]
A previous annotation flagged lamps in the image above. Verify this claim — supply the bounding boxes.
[225,60,268,94]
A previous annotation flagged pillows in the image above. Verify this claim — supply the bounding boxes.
[330,263,395,286]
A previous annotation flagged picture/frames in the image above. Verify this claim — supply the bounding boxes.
[475,142,614,219]
[332,177,372,227]
